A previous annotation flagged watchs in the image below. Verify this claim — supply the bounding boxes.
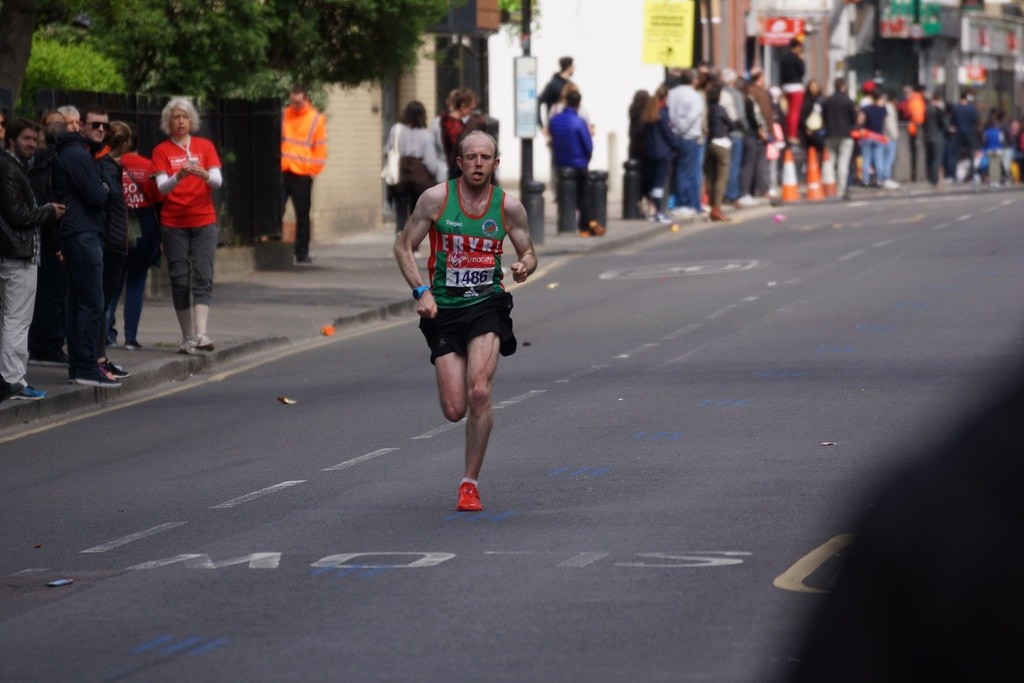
[412,285,430,300]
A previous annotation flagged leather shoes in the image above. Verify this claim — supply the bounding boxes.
[0,383,24,403]
[710,205,731,222]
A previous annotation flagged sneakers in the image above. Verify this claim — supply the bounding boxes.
[68,359,131,388]
[455,482,482,511]
[175,332,214,355]
[9,385,47,401]
[124,340,143,351]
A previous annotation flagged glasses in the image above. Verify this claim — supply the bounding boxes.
[85,121,110,132]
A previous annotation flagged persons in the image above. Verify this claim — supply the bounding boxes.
[780,38,806,93]
[382,87,499,259]
[536,57,606,239]
[254,86,328,263]
[629,64,1024,222]
[0,97,222,399]
[393,131,537,513]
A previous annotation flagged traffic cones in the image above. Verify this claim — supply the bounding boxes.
[803,147,828,204]
[771,149,805,206]
[820,148,844,203]
